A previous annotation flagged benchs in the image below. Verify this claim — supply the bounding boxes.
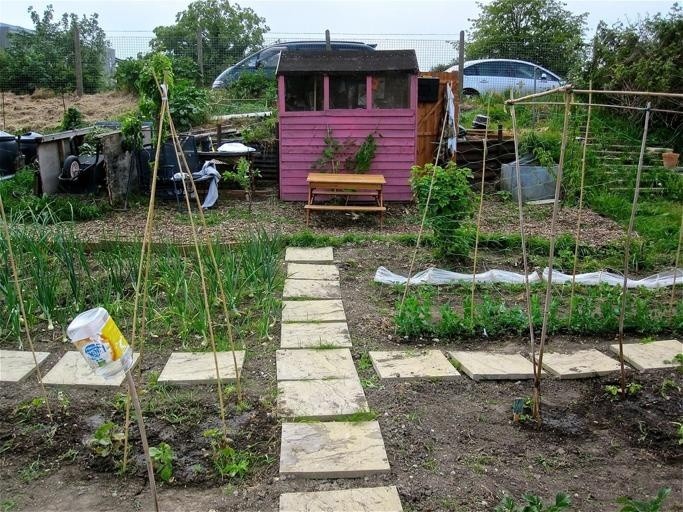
[303,191,385,233]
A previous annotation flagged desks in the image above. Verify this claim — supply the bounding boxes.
[305,173,385,233]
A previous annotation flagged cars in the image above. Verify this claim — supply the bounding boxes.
[443,58,568,101]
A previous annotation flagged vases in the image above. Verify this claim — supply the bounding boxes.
[661,152,680,170]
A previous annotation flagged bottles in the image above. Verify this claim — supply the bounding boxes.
[68,306,132,381]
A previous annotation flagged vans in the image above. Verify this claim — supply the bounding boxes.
[212,40,378,90]
